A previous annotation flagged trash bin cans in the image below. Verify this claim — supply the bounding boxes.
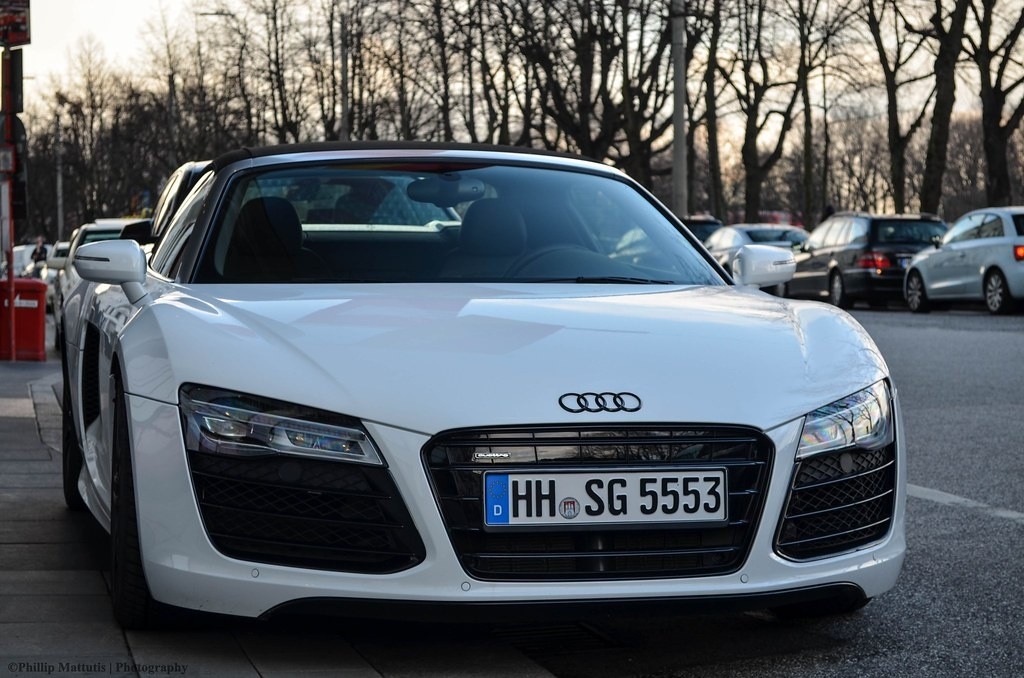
[0,278,48,363]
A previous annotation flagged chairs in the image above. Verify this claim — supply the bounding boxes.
[429,197,529,281]
[225,195,329,282]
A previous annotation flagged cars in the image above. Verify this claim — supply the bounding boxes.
[775,208,946,312]
[54,142,906,636]
[1,141,463,307]
[703,222,809,300]
[603,214,723,271]
[903,204,1024,315]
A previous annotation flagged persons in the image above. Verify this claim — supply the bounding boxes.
[819,205,836,223]
[30,237,47,278]
